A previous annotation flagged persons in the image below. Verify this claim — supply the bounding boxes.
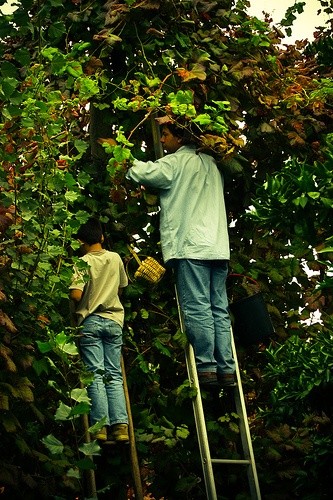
[96,118,237,386]
[67,216,130,441]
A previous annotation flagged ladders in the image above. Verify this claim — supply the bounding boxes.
[66,352,143,500]
[174,279,262,500]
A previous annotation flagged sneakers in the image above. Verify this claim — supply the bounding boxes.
[107,423,129,441]
[220,374,235,385]
[90,426,108,440]
[197,371,219,384]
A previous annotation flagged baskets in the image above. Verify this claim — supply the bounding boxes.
[125,243,164,285]
